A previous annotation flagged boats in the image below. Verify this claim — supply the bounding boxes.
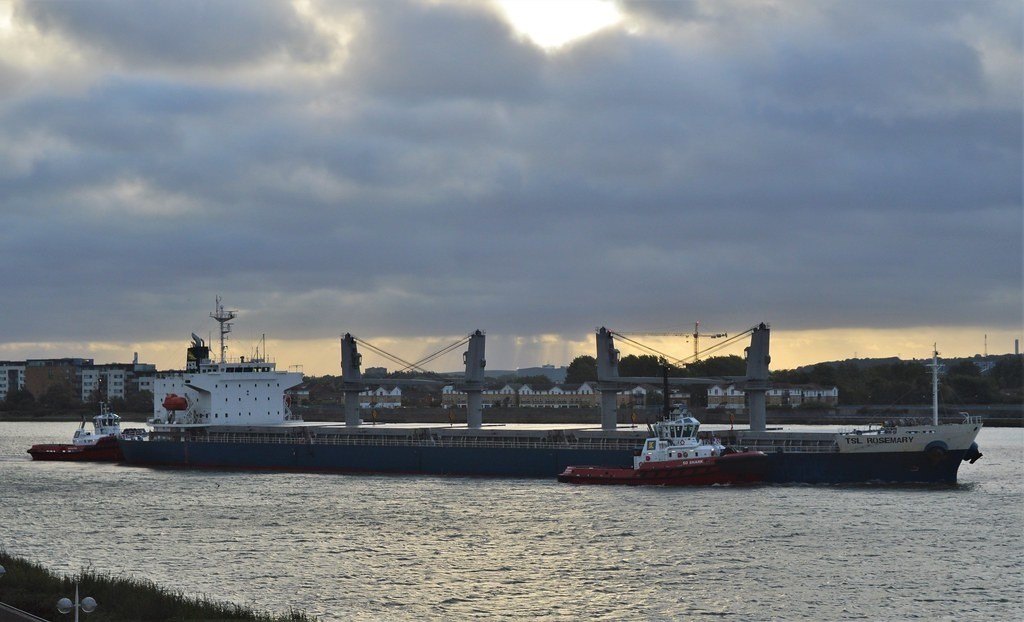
[112,293,985,488]
[558,407,769,485]
[27,399,149,462]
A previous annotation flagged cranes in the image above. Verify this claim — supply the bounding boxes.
[613,319,729,363]
